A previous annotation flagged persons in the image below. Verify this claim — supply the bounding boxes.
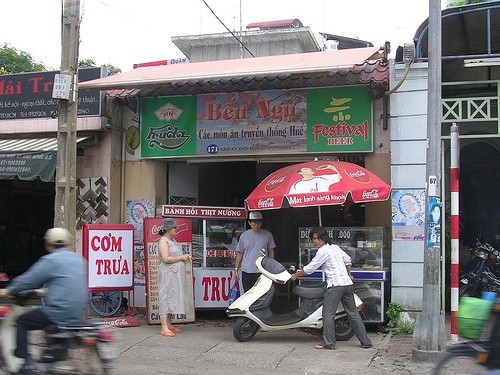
[291,226,373,350]
[235,212,276,293]
[431,203,441,224]
[0,228,89,358]
[156,218,193,337]
[289,164,342,194]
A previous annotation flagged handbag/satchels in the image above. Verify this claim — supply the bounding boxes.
[229,289,240,306]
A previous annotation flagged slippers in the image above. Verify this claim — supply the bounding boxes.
[315,341,339,349]
[362,342,374,349]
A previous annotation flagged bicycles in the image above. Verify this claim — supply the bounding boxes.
[40,282,123,317]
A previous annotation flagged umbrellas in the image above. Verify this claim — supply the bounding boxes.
[245,157,391,283]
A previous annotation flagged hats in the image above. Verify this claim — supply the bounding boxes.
[43,227,71,245]
[248,211,264,221]
[160,218,180,232]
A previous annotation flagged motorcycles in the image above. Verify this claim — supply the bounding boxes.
[431,302,500,375]
[447,233,500,306]
[226,249,364,341]
[0,289,118,375]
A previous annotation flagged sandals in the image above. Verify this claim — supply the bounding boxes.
[161,331,175,337]
[170,326,182,333]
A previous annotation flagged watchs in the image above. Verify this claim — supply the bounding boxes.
[235,268,240,271]
